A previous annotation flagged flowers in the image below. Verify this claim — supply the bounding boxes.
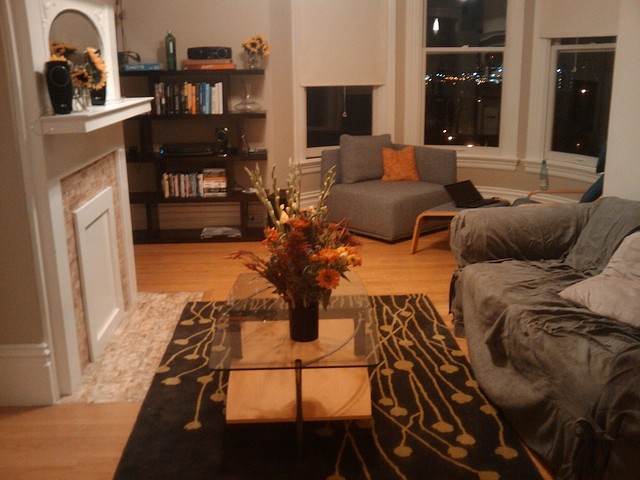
[244,35,270,58]
[221,157,362,311]
[72,48,107,90]
[51,40,77,66]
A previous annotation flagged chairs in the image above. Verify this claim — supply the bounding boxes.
[413,142,607,255]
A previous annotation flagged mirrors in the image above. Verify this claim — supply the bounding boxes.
[49,10,105,69]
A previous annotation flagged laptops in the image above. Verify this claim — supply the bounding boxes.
[442,179,501,209]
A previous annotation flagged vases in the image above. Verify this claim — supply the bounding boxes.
[234,81,261,112]
[71,86,91,113]
[290,300,320,341]
[247,53,262,70]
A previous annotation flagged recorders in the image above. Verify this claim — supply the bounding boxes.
[187,46,232,60]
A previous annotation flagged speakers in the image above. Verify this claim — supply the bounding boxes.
[89,87,106,106]
[45,61,73,114]
[214,127,228,156]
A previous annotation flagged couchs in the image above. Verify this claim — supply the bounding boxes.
[321,144,457,241]
[449,196,640,480]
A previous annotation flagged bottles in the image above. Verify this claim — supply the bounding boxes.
[165,28,177,70]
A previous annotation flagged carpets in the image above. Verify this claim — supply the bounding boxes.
[114,294,546,480]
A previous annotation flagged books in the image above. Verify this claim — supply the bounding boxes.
[154,82,224,115]
[124,63,166,70]
[163,173,203,198]
[204,169,227,198]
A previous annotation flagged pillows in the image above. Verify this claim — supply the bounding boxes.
[560,232,640,329]
[339,133,393,182]
[382,146,420,181]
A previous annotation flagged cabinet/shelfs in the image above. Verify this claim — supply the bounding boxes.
[121,68,286,244]
